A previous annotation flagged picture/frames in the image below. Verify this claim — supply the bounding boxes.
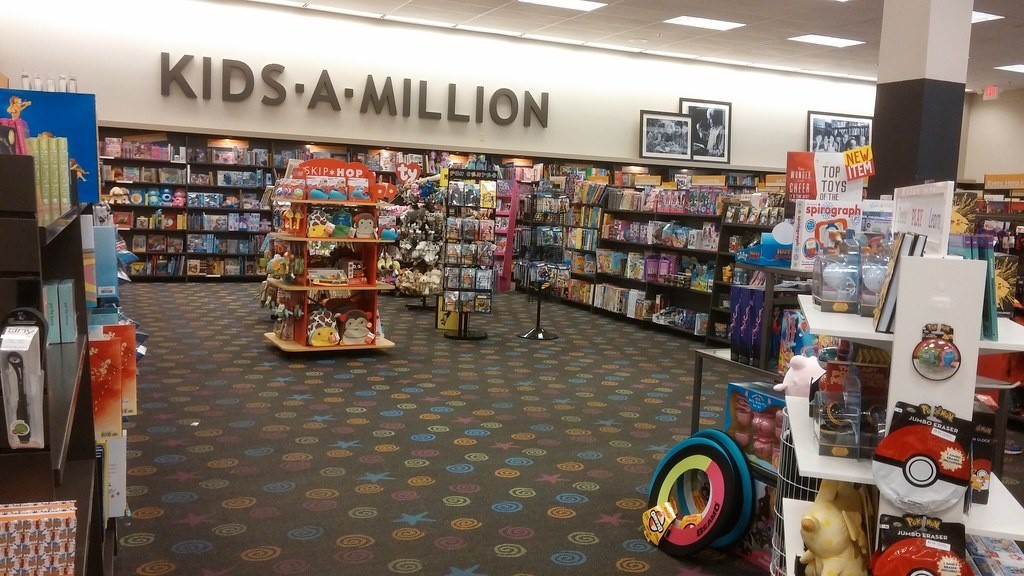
[806,110,873,155]
[639,110,693,160]
[679,97,732,165]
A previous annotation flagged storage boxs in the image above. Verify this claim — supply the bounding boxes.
[87,323,138,519]
[789,198,863,272]
[44,279,77,346]
[729,285,812,375]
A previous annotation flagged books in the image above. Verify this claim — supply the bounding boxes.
[948,235,998,342]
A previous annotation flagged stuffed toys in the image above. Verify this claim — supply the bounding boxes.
[799,479,873,576]
[378,180,441,295]
[266,207,376,346]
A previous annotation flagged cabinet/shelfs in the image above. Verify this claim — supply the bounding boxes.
[688,261,824,576]
[0,154,116,576]
[946,175,1024,327]
[263,159,398,353]
[97,120,788,357]
[783,295,1024,576]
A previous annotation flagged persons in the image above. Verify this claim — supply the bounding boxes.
[247,265,253,273]
[189,264,197,274]
[812,127,866,153]
[646,121,687,154]
[697,108,725,156]
